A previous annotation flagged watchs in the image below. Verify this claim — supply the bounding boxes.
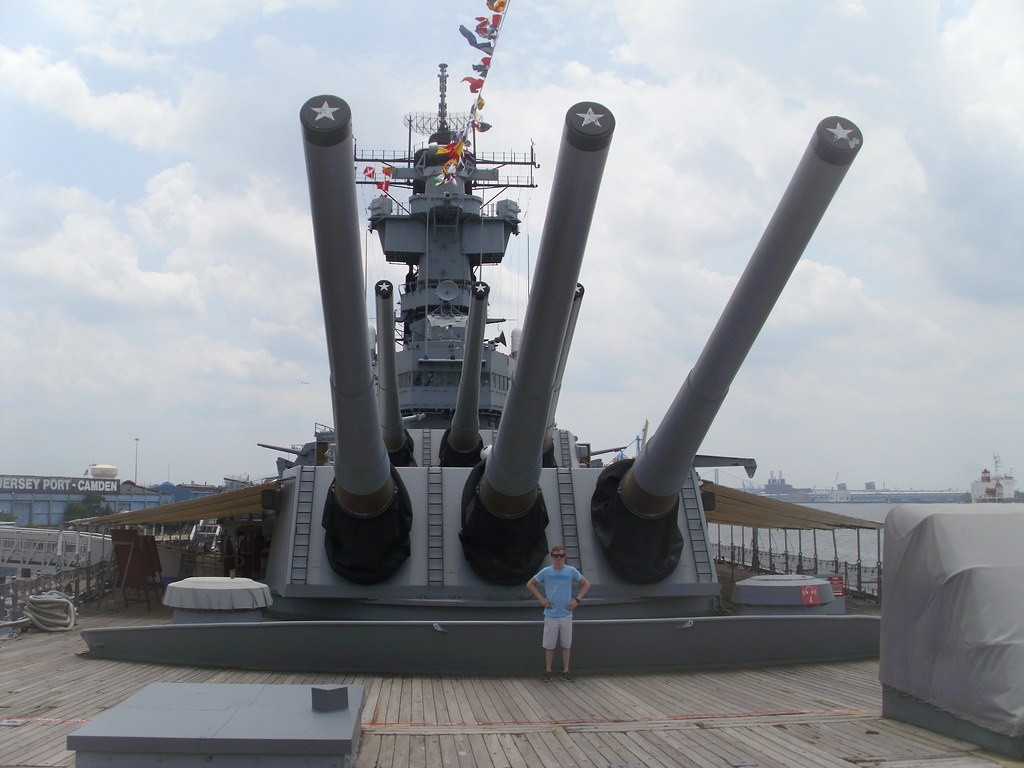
[575,598,581,603]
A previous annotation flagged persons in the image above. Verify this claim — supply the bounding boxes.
[527,544,590,683]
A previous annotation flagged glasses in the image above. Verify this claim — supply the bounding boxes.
[551,554,564,559]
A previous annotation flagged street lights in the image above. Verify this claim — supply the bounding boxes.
[134,437,140,484]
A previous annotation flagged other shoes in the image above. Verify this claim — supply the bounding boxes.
[543,671,553,682]
[562,672,576,682]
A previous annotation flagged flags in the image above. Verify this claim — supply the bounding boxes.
[363,166,375,179]
[377,180,390,191]
[382,167,392,177]
